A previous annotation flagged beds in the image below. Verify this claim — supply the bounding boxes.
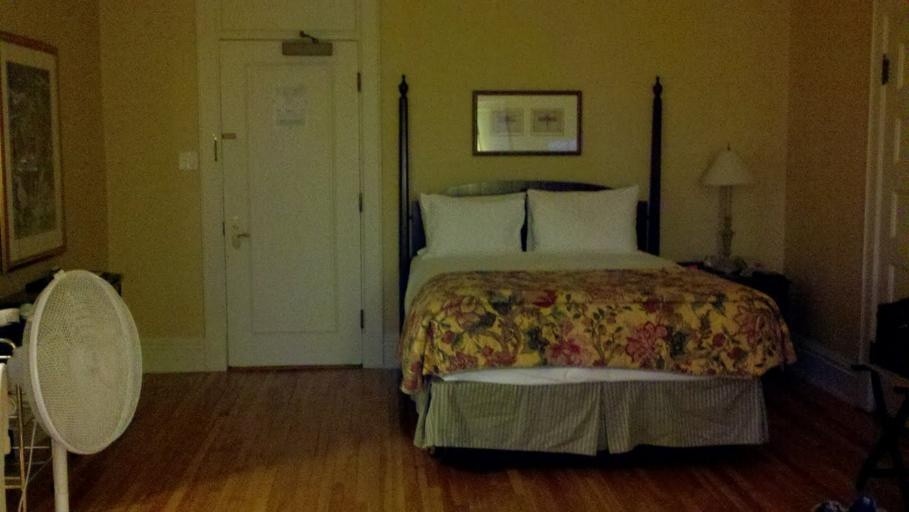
[389,67,805,478]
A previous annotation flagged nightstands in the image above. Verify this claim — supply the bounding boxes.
[678,264,791,384]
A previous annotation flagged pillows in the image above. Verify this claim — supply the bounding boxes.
[415,188,526,260]
[523,187,641,258]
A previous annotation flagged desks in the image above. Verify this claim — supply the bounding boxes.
[853,356,908,511]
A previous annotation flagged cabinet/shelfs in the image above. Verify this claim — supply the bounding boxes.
[0,270,123,364]
[0,338,65,511]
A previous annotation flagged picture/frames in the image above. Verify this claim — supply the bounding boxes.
[0,29,67,272]
[471,85,584,158]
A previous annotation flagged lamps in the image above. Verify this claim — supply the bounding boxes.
[695,144,756,272]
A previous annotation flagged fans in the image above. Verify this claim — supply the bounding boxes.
[8,269,144,512]
